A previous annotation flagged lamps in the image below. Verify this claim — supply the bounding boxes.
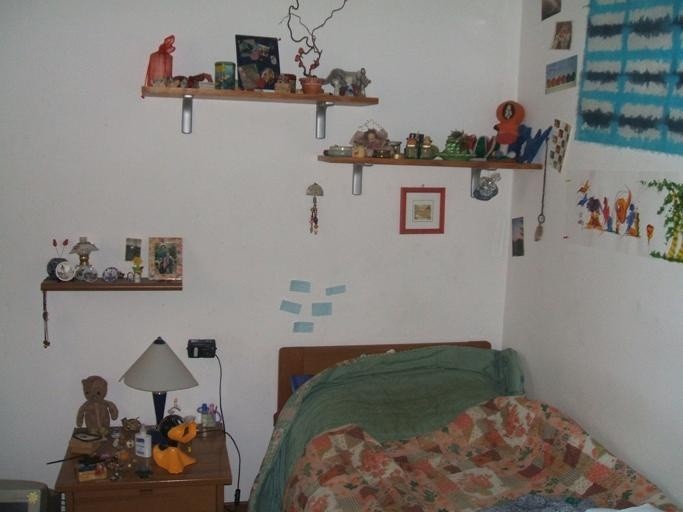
[118,336,199,444]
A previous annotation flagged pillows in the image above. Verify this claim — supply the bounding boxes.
[290,376,314,391]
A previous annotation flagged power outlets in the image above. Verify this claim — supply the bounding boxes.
[188,340,215,359]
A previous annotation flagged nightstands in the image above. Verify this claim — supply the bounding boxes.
[55,426,232,511]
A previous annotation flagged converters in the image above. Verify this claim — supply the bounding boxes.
[186,338,216,360]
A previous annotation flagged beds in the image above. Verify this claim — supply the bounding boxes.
[247,341,683,512]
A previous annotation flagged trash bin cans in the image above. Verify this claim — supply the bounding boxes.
[0,480,49,511]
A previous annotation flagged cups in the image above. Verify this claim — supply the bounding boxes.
[201,413,217,428]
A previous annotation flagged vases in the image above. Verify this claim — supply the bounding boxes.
[298,78,321,93]
[133,272,142,283]
[47,258,68,278]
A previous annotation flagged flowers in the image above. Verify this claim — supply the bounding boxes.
[278,0,347,77]
[52,239,68,258]
[131,256,143,275]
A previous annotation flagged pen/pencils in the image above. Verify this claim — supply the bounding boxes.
[202,403,216,413]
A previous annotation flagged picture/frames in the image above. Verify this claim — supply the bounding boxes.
[148,237,183,281]
[235,34,280,90]
[400,187,445,234]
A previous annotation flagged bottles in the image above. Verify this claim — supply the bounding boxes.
[420,139,433,158]
[404,139,418,159]
[134,424,153,458]
